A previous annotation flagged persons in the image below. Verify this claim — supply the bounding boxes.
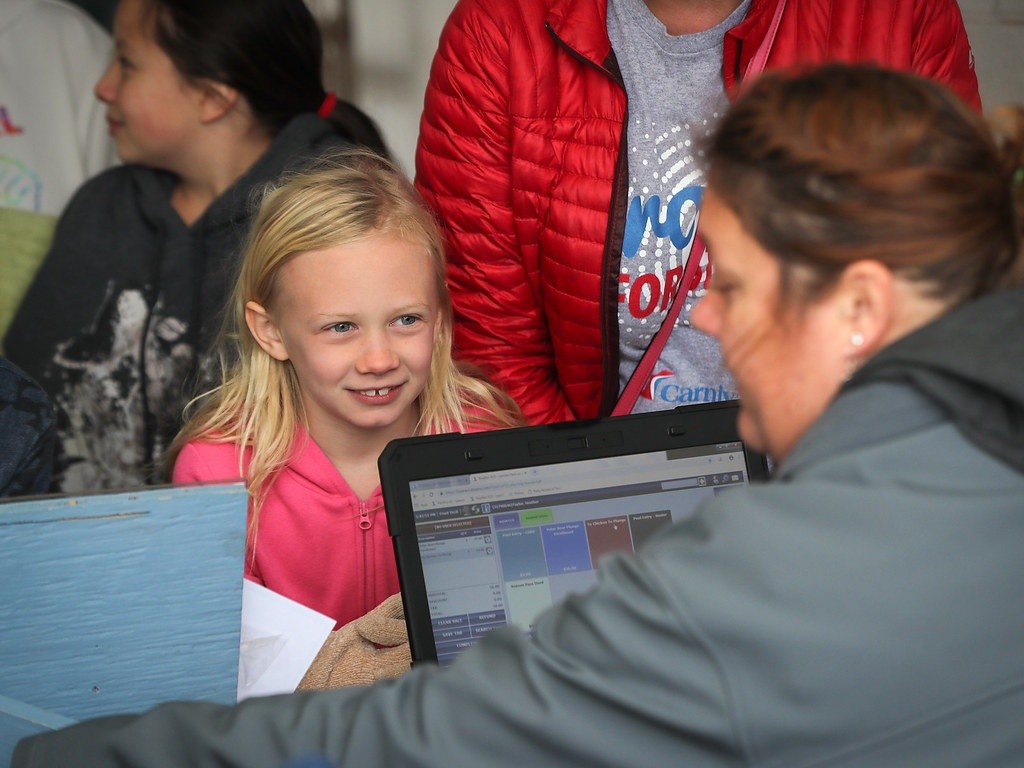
[172,147,528,705]
[0,0,391,503]
[413,0,982,434]
[12,61,1024,768]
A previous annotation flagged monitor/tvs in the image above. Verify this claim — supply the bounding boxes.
[379,397,771,672]
